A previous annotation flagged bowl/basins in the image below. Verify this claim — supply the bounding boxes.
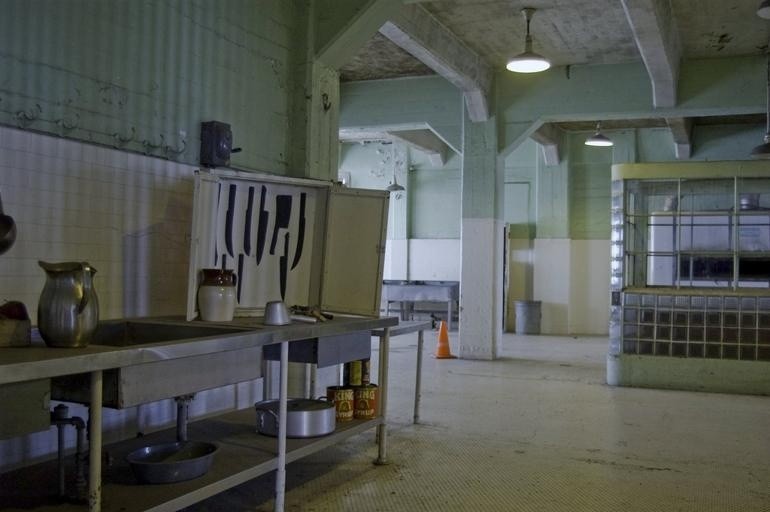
[128,441,221,482]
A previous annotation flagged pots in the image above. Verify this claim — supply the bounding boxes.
[0,195,16,256]
[252,395,338,436]
[263,301,317,327]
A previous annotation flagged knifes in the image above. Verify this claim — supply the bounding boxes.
[225,183,309,301]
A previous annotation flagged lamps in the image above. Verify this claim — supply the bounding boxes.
[584,121,614,147]
[506,8,550,73]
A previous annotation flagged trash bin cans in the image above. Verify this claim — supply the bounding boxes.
[513,301,541,335]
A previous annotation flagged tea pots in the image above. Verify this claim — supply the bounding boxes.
[36,259,102,348]
[198,269,238,324]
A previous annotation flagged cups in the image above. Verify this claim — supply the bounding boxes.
[738,191,760,210]
